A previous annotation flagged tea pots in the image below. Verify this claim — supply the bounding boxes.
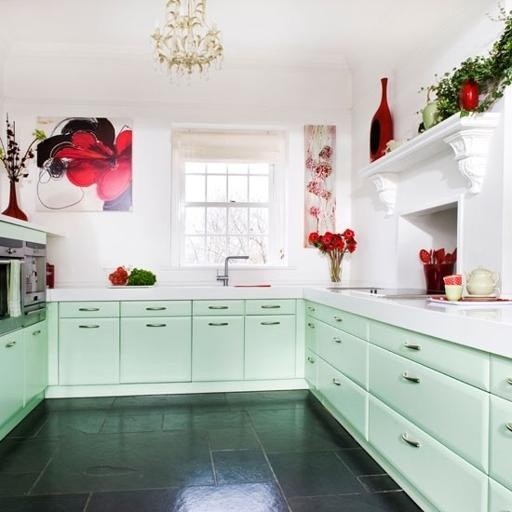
[417,86,446,131]
[465,267,500,294]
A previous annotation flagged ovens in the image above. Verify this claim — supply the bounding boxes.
[0,236,49,336]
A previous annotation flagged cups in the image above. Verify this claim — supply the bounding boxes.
[443,274,462,285]
[445,284,463,301]
[423,263,454,294]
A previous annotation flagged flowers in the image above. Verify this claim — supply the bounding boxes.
[0,112,46,181]
[308,230,356,282]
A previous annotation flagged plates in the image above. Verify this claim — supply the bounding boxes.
[464,294,497,298]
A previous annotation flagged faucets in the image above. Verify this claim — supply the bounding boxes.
[224,256,249,286]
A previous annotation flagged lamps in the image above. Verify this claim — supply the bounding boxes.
[152,0,223,87]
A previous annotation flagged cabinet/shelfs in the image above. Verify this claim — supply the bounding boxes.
[302,300,318,399]
[369,320,490,512]
[58,299,297,398]
[0,317,48,441]
[490,356,512,512]
[320,304,370,453]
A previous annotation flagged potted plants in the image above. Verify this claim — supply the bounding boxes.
[419,248,457,294]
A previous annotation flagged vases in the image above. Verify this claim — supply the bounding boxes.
[327,257,343,286]
[418,9,512,134]
[1,182,28,221]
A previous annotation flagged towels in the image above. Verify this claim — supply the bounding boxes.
[7,259,22,317]
